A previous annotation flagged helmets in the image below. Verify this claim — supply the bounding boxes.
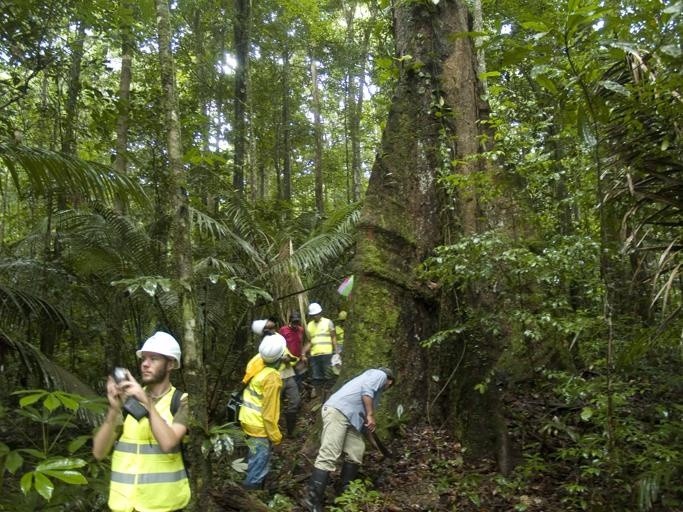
[308,302,322,316]
[336,310,347,321]
[135,330,181,371]
[250,319,268,336]
[258,331,287,363]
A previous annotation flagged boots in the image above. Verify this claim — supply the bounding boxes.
[304,466,329,512]
[285,412,298,437]
[333,461,360,496]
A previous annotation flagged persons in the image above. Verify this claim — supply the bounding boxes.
[305,303,342,400]
[334,310,347,354]
[93,330,191,512]
[298,367,396,512]
[251,319,300,439]
[238,334,287,489]
[280,313,312,375]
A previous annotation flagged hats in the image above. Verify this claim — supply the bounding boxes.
[291,311,300,322]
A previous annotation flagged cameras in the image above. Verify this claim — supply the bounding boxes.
[111,366,127,384]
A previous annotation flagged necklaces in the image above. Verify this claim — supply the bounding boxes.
[148,382,172,400]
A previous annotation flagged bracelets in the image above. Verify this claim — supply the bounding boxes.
[300,353,305,356]
[105,420,115,426]
